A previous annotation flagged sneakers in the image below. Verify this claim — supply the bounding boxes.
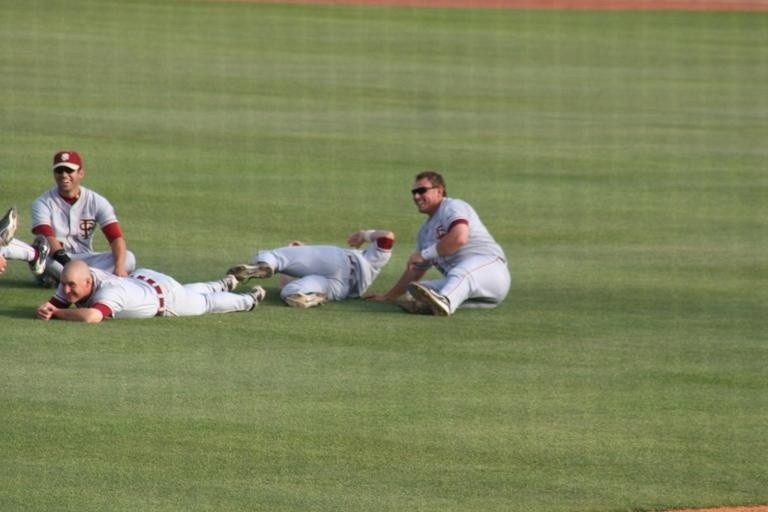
[0,206,18,247]
[247,284,267,312]
[408,281,451,317]
[286,292,329,308]
[29,234,51,276]
[226,263,275,285]
[223,274,239,292]
[414,300,436,316]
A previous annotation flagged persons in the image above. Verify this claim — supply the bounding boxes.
[29,151,137,288]
[0,206,49,275]
[361,171,514,319]
[36,259,266,324]
[227,229,395,309]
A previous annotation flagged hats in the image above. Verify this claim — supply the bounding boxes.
[53,151,82,171]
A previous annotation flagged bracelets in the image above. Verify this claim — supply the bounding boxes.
[364,230,375,244]
[421,243,438,262]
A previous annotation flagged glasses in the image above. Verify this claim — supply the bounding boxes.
[411,185,436,195]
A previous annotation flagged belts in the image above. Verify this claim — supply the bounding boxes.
[130,274,164,317]
[349,255,355,292]
